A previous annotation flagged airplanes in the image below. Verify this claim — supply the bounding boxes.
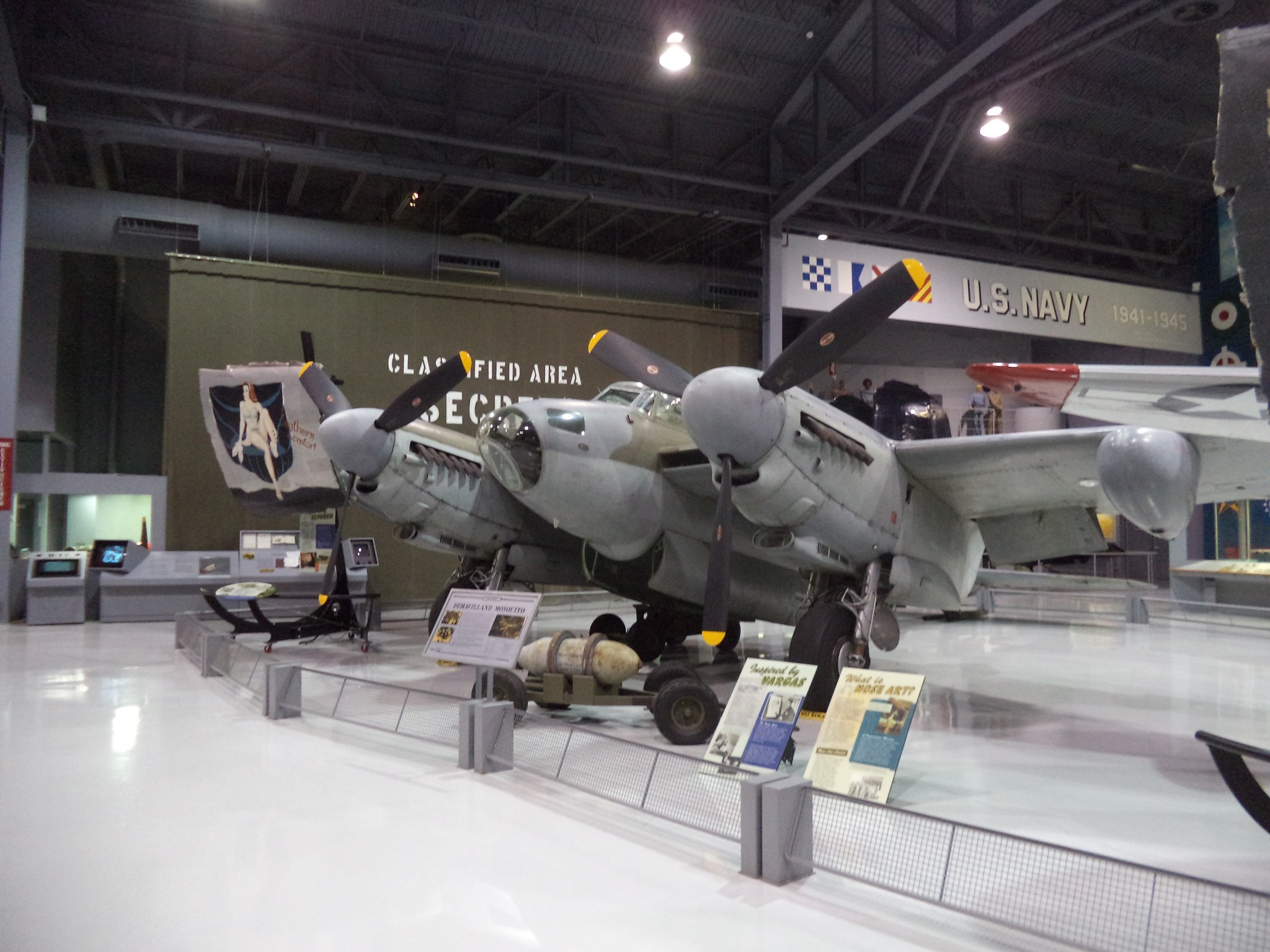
[297,259,1270,701]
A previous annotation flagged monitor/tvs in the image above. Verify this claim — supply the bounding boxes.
[351,541,375,565]
[100,544,126,566]
